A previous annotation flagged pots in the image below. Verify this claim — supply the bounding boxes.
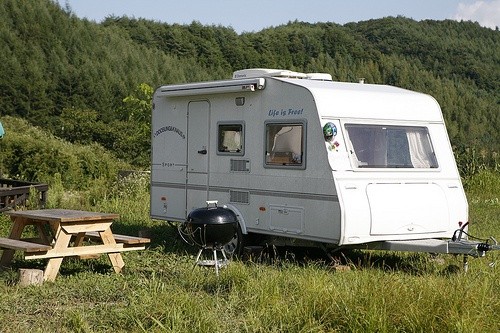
[184,200,239,249]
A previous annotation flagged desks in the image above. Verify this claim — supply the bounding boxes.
[1,208,125,283]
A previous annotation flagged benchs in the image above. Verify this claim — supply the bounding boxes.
[85,232,151,250]
[0,238,52,259]
